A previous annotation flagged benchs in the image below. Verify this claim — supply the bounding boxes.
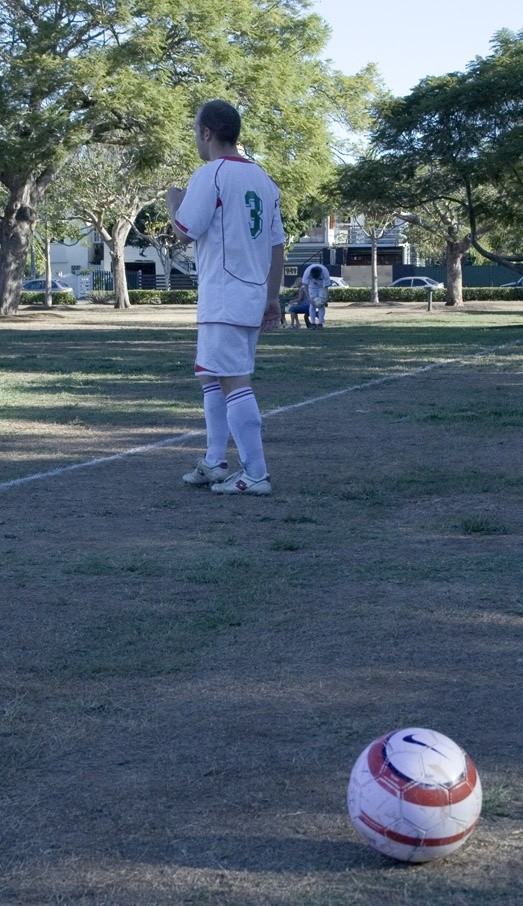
[280,297,329,329]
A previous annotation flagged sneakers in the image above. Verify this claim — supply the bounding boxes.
[182,453,229,488]
[210,459,273,496]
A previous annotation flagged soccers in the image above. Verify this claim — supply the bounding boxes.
[347,728,482,863]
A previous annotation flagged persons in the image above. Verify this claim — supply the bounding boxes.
[286,277,311,330]
[166,101,285,497]
[302,264,330,330]
